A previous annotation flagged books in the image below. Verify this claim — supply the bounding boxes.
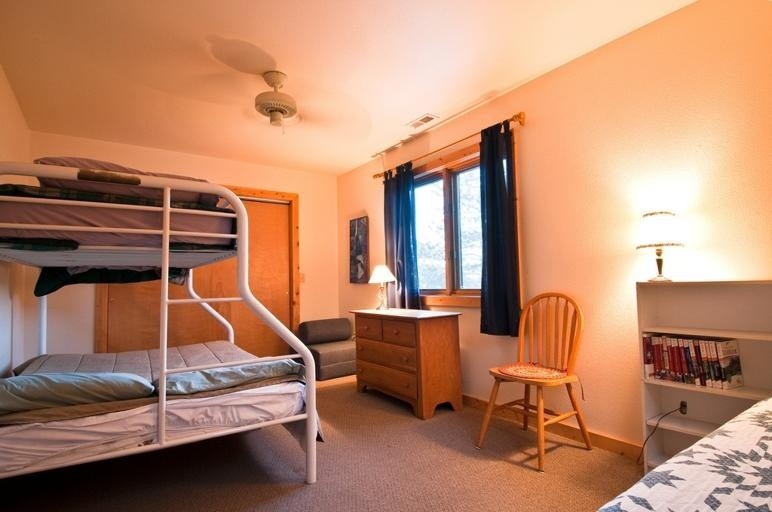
[641,331,745,390]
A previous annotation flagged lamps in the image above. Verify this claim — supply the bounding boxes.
[634,210,688,284]
[368,263,398,311]
[254,70,298,128]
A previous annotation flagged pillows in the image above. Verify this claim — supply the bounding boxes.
[150,358,307,396]
[33,156,220,207]
[0,372,156,416]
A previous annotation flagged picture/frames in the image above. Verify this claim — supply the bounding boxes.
[348,215,370,285]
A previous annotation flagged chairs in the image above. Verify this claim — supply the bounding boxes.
[474,291,594,473]
[299,317,357,381]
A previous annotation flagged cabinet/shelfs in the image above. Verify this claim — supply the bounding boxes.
[635,279,772,475]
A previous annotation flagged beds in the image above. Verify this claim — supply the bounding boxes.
[0,161,316,484]
[592,397,772,512]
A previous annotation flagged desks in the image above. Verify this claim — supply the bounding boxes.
[349,307,464,421]
